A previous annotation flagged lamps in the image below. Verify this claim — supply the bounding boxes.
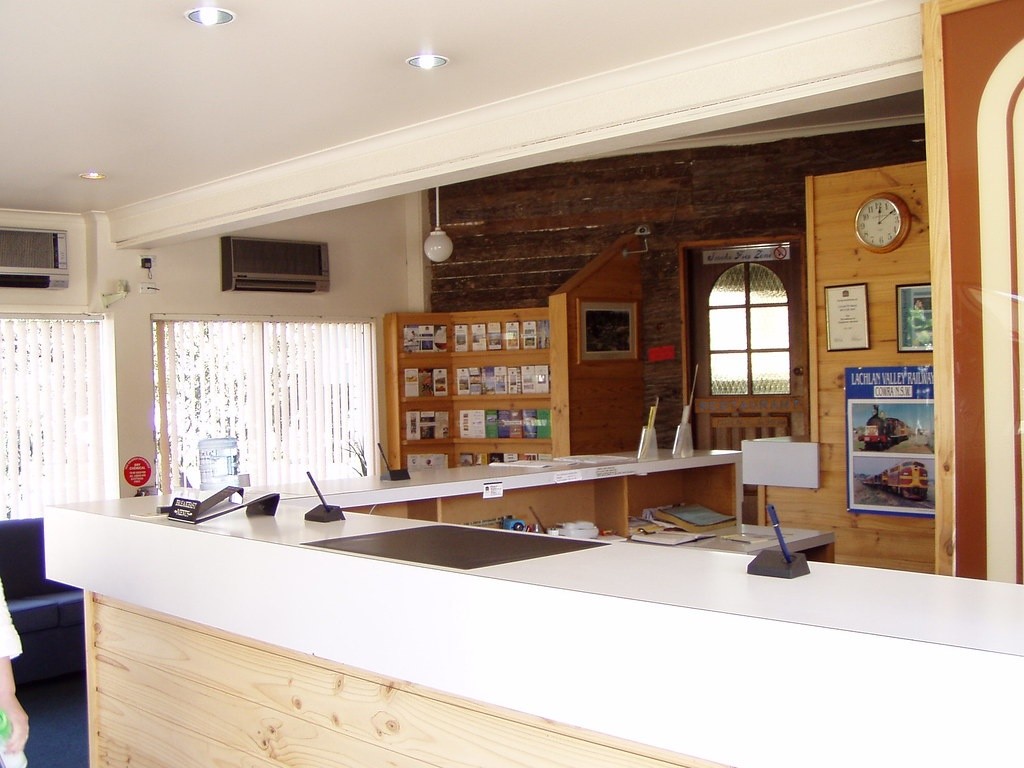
[406,54,449,70]
[423,187,453,262]
[185,7,236,27]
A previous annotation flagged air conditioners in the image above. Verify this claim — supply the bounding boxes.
[220,236,331,296]
[0,226,69,291]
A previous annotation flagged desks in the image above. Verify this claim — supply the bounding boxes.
[687,524,835,562]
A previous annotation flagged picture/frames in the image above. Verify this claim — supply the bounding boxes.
[895,283,933,353]
[576,297,642,365]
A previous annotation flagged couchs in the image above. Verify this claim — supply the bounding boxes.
[0,519,86,687]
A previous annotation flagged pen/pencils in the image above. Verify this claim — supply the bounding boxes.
[529,506,547,533]
[377,442,391,471]
[767,504,792,564]
[522,523,539,532]
[306,471,330,511]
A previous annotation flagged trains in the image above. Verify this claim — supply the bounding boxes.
[860,404,911,450]
[860,461,928,501]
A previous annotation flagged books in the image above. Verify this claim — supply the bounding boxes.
[403,319,553,470]
[631,531,716,545]
[720,532,792,544]
[653,504,737,531]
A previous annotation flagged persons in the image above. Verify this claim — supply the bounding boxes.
[0,575,29,768]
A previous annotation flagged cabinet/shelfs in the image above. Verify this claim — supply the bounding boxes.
[378,293,569,476]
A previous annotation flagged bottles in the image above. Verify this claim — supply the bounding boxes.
[0,708,27,768]
[199,438,239,490]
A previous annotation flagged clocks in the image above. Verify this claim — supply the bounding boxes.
[854,198,901,248]
